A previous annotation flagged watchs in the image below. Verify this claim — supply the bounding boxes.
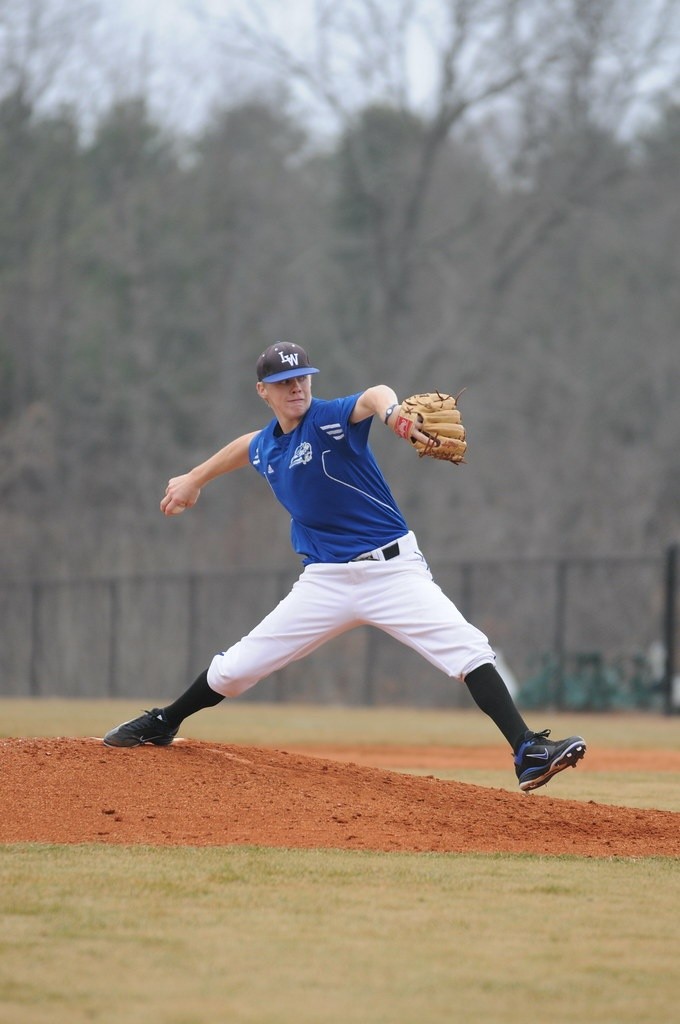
[384,403,399,425]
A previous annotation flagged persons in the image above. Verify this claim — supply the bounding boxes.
[103,341,586,790]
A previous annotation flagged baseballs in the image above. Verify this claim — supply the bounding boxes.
[171,505,185,514]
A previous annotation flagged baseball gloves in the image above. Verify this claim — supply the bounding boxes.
[393,392,468,465]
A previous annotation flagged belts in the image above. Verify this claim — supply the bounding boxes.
[362,543,401,563]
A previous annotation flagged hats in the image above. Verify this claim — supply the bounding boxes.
[255,341,319,385]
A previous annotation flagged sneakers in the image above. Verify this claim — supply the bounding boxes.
[104,707,179,748]
[513,730,586,791]
